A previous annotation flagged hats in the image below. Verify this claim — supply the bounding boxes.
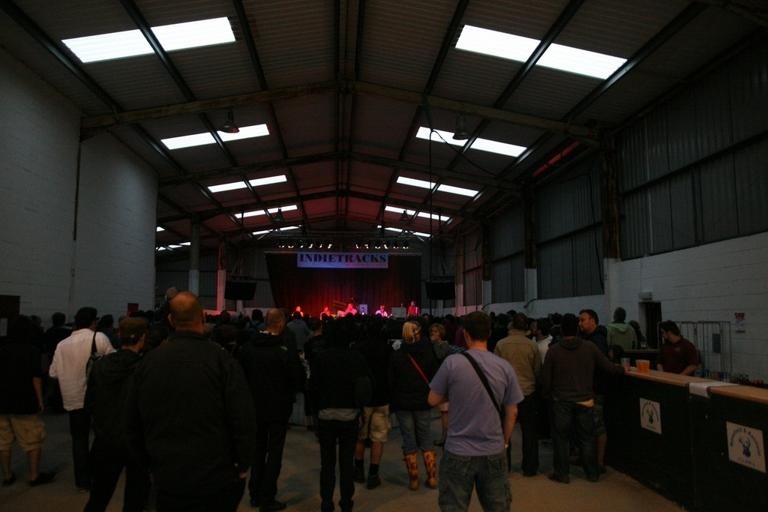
[118,317,148,340]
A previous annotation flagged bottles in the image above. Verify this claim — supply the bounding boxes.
[703,370,764,388]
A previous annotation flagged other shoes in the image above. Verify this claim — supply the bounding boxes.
[571,459,591,468]
[588,466,606,481]
[368,471,381,489]
[523,470,535,476]
[4,476,16,487]
[570,447,576,456]
[250,496,286,511]
[549,472,570,483]
[30,473,55,486]
[75,481,90,489]
[352,466,365,482]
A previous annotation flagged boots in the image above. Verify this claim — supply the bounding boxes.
[404,450,440,490]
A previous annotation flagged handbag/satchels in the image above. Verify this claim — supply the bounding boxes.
[85,331,103,378]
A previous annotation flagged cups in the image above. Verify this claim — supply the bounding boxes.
[621,358,632,372]
[657,364,664,375]
